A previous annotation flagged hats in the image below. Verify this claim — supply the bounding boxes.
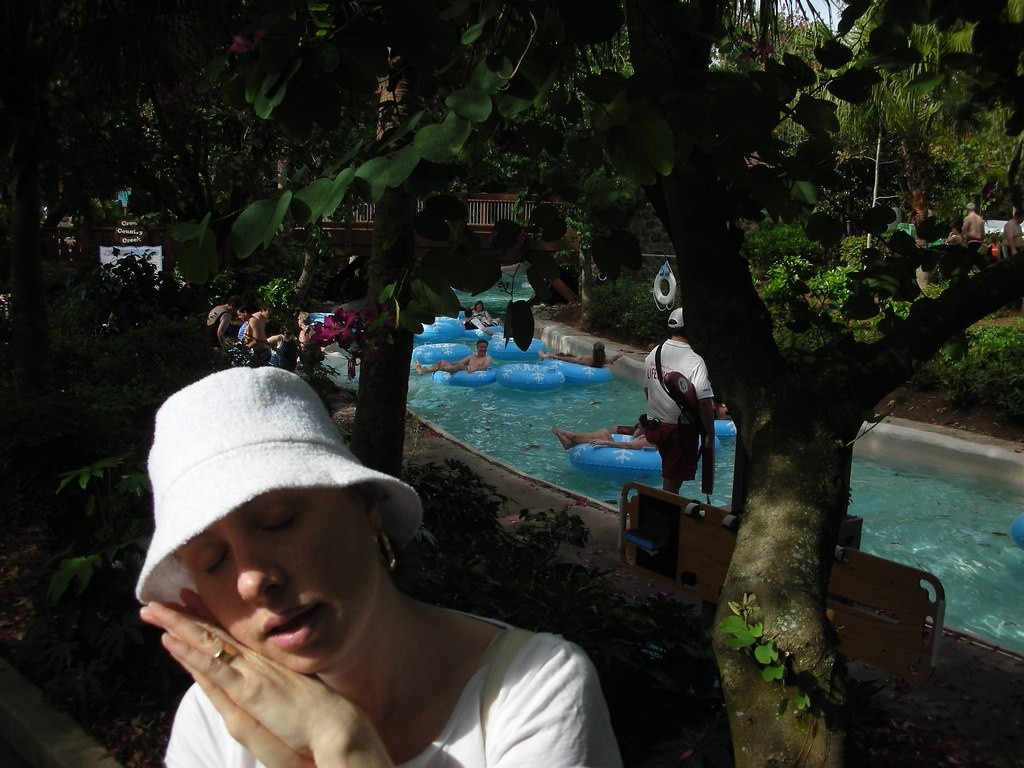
[133,365,422,607]
[668,308,684,328]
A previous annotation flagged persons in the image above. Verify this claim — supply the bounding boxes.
[134,365,624,768]
[461,301,504,334]
[207,299,325,370]
[538,341,623,368]
[415,339,492,375]
[551,306,733,495]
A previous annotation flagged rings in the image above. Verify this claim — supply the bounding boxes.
[216,643,238,662]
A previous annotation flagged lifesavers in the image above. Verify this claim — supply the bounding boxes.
[494,362,566,395]
[697,433,721,456]
[413,310,505,348]
[567,433,664,480]
[713,418,740,438]
[431,368,496,387]
[412,342,474,366]
[1008,511,1024,552]
[486,336,548,361]
[330,359,421,398]
[536,358,613,384]
